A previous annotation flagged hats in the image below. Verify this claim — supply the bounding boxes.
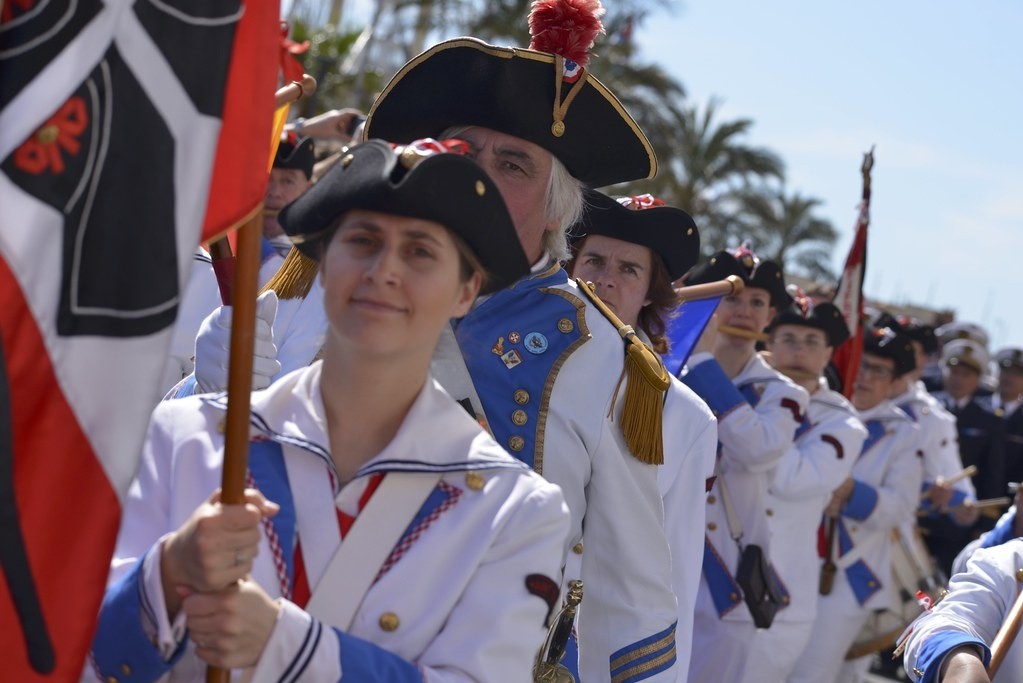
[765,281,850,348]
[276,132,531,297]
[895,313,938,358]
[863,310,917,377]
[683,239,760,285]
[742,256,794,310]
[990,348,1023,372]
[271,128,316,176]
[566,183,701,284]
[362,0,657,190]
[932,321,988,373]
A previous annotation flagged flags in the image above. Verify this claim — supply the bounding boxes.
[0,1,278,683]
[829,198,869,400]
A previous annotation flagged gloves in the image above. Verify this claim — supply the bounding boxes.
[195,290,283,393]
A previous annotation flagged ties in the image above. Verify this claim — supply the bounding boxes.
[996,403,1005,417]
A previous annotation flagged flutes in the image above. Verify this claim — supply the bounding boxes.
[654,273,743,302]
[774,366,820,379]
[717,323,773,342]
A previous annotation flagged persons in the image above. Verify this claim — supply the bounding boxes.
[173,108,368,379]
[164,37,679,682]
[83,137,571,683]
[658,247,1023,683]
[562,190,718,682]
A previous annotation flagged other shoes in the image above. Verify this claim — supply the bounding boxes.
[870,647,908,679]
